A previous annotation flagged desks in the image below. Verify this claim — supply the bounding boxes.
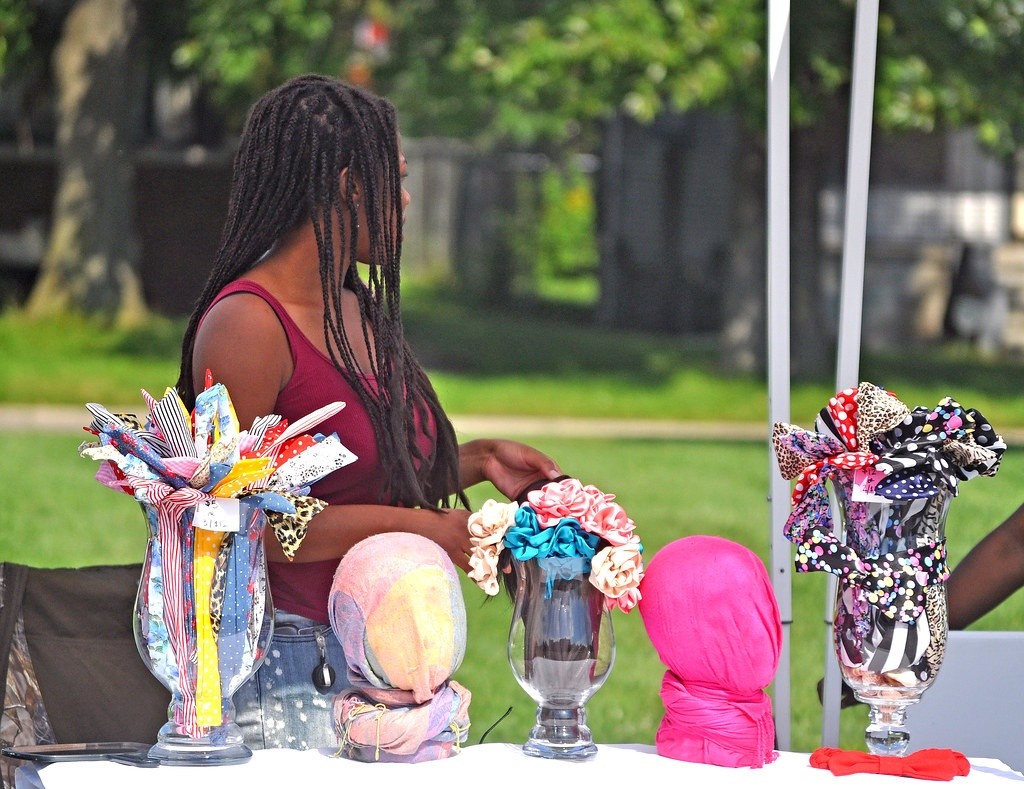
[0,743,1024,789]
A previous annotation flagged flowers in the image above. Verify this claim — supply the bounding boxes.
[467,474,645,616]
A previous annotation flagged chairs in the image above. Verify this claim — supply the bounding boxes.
[0,561,171,742]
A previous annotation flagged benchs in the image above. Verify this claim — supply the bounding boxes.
[907,631,1024,774]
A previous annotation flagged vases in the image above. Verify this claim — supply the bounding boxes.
[831,488,954,756]
[507,558,615,759]
[133,503,275,762]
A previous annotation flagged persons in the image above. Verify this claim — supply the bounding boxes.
[173,72,562,749]
[817,502,1024,711]
